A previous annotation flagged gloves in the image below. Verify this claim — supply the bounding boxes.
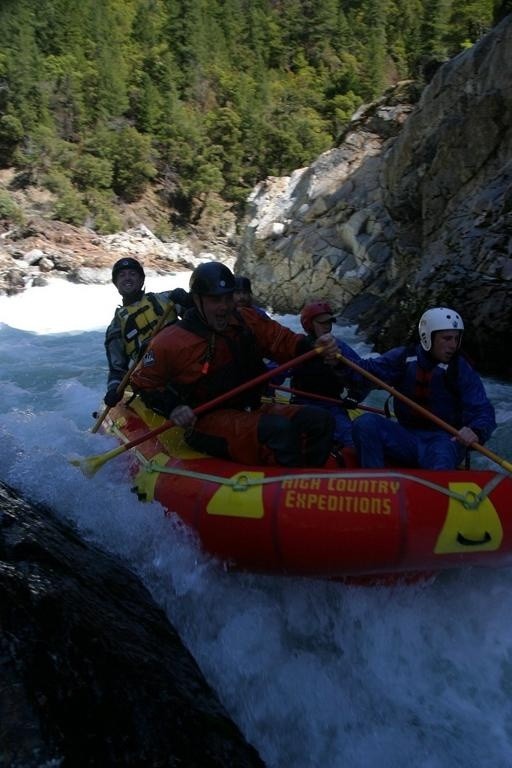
[103,383,123,406]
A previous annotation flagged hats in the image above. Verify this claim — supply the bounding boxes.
[301,302,333,332]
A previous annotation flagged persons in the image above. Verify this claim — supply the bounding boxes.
[233,269,287,384]
[104,257,193,406]
[326,308,497,472]
[263,302,373,446]
[129,263,339,467]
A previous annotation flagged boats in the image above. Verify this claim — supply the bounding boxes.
[97,389,511,586]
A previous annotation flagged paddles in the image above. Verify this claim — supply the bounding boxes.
[67,339,340,480]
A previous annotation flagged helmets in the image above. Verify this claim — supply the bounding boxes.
[417,307,466,351]
[235,277,252,292]
[112,258,145,281]
[190,262,235,295]
